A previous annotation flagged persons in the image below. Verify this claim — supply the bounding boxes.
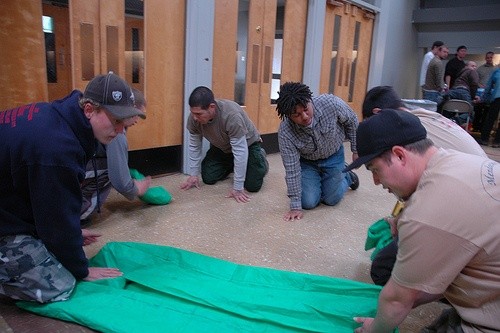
[275,81,359,222]
[176,86,269,204]
[342,108,500,333]
[362,86,490,287]
[418,40,500,148]
[0,73,148,304]
[79,86,150,229]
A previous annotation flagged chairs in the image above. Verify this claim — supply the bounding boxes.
[441,99,472,131]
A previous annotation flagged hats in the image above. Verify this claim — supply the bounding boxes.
[82,71,148,121]
[341,108,428,173]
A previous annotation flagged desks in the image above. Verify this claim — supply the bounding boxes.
[470,101,500,146]
